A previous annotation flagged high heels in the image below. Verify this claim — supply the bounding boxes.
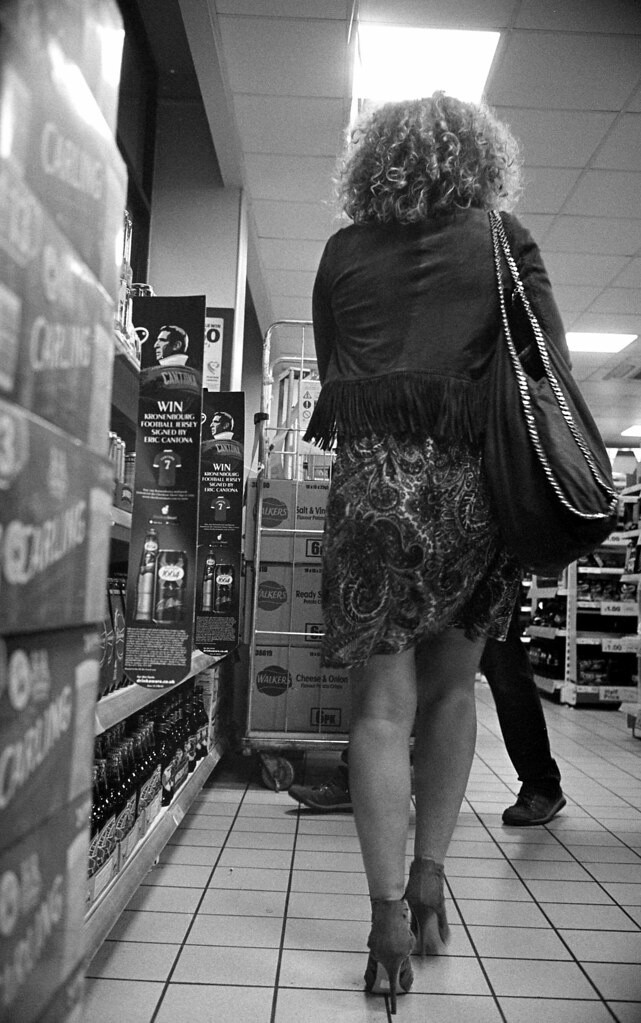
[403,858,451,959]
[364,897,414,1014]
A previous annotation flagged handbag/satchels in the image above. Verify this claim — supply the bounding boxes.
[487,207,625,578]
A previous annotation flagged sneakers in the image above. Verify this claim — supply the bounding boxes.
[501,788,566,824]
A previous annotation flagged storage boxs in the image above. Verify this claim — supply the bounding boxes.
[0,0,350,1023]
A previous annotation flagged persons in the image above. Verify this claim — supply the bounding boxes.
[288,615,566,824]
[141,325,200,418]
[202,412,244,473]
[302,93,571,1014]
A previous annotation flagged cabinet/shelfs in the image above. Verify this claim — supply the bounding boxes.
[83,651,231,972]
[475,516,641,740]
